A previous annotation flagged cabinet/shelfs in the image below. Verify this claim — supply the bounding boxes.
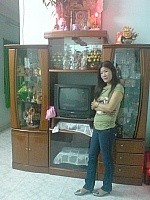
[3,30,150,187]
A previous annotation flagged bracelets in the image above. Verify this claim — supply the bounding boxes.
[97,104,99,107]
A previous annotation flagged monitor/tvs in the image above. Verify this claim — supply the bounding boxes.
[54,84,97,128]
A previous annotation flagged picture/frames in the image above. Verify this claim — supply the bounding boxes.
[70,8,90,30]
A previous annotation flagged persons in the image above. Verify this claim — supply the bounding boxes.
[73,61,124,196]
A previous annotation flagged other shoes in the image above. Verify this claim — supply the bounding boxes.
[93,189,110,196]
[74,189,92,195]
